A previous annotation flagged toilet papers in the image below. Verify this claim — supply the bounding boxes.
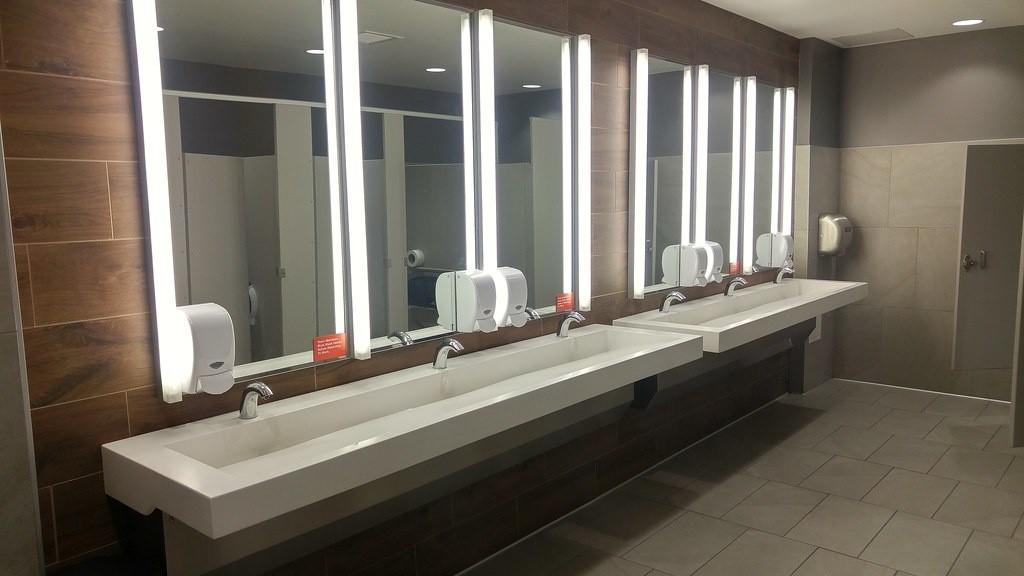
[406,248,423,268]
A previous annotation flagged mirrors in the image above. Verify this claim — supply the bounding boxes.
[121,0,799,407]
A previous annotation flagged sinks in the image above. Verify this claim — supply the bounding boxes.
[650,278,867,353]
[174,323,702,540]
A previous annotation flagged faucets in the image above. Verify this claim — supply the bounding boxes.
[526,306,540,320]
[434,337,466,370]
[753,264,760,272]
[241,381,272,418]
[662,291,686,312]
[777,267,795,285]
[388,327,416,345]
[725,277,748,297]
[559,311,585,338]
